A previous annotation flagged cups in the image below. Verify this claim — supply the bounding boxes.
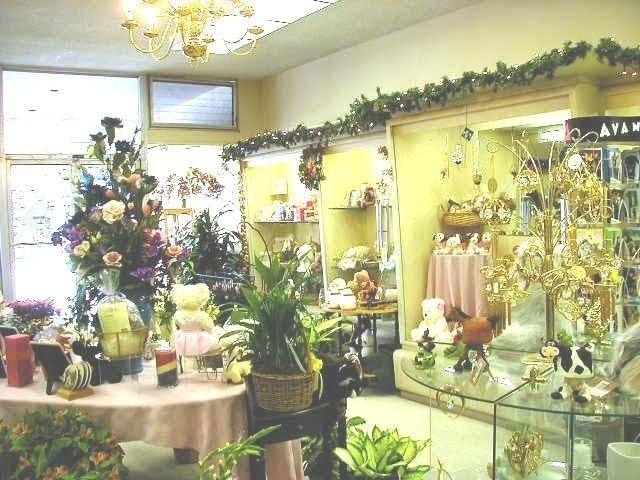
[605,439,640,480]
[154,348,178,388]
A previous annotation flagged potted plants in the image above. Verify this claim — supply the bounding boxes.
[222,219,359,413]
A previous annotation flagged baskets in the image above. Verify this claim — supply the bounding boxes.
[443,210,484,228]
[251,369,316,413]
[101,327,149,358]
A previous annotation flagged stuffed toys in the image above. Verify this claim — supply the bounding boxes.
[171,283,214,356]
[431,232,491,255]
[347,270,369,299]
[411,298,452,343]
[446,304,498,370]
[551,344,592,403]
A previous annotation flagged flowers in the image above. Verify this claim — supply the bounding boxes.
[0,405,129,480]
[49,112,191,345]
[6,296,59,340]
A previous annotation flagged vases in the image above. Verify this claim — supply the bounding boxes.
[93,328,150,374]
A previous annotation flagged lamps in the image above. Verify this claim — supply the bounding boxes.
[116,0,264,62]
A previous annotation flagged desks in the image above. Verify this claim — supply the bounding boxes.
[1,366,303,480]
[323,300,399,396]
[431,253,492,318]
